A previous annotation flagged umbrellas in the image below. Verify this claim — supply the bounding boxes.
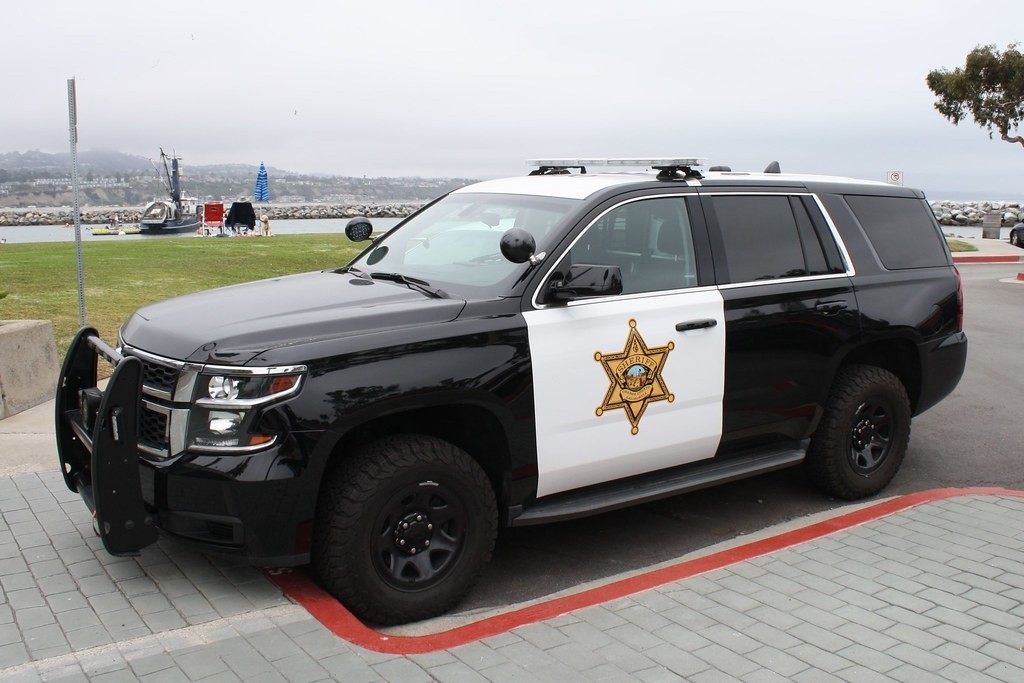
[254,160,270,222]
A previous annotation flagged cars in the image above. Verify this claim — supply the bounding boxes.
[1009,219,1024,247]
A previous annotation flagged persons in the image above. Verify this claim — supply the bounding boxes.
[235,196,252,235]
[109,212,119,228]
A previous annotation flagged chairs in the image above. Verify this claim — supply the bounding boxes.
[225,202,256,235]
[200,201,224,237]
[625,218,686,292]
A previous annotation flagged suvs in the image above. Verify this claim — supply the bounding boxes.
[52,155,969,630]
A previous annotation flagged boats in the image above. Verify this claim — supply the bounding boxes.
[136,145,204,236]
[86,213,141,236]
